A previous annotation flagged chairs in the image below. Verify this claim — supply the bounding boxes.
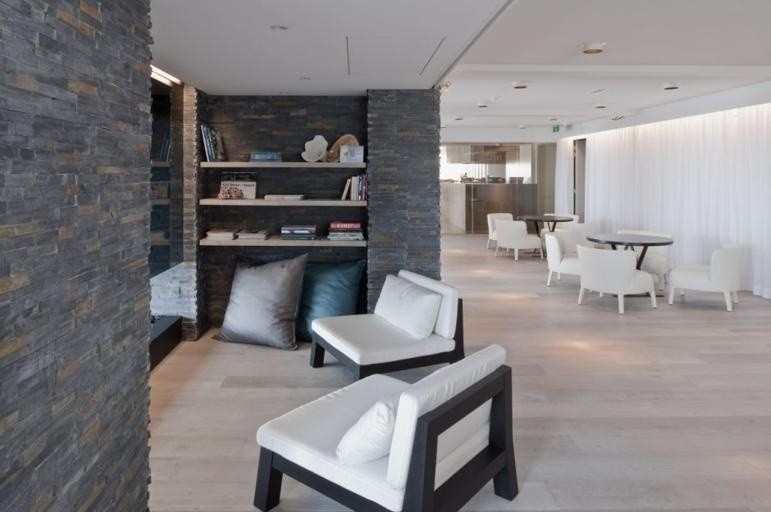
[669,244,743,311]
[541,213,579,245]
[576,243,657,314]
[545,230,581,286]
[494,220,544,260]
[484,213,512,248]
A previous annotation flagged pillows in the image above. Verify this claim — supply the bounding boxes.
[373,274,442,340]
[335,385,410,467]
[212,254,308,351]
[296,260,366,343]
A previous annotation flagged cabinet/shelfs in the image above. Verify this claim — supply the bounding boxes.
[439,182,536,234]
[198,162,367,247]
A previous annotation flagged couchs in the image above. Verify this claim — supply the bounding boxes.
[309,269,465,379]
[252,344,517,512]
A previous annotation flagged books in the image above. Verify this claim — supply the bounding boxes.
[207,227,236,240]
[264,192,306,200]
[343,175,369,202]
[237,226,270,241]
[156,132,171,161]
[327,219,363,241]
[280,224,318,241]
[199,124,224,162]
